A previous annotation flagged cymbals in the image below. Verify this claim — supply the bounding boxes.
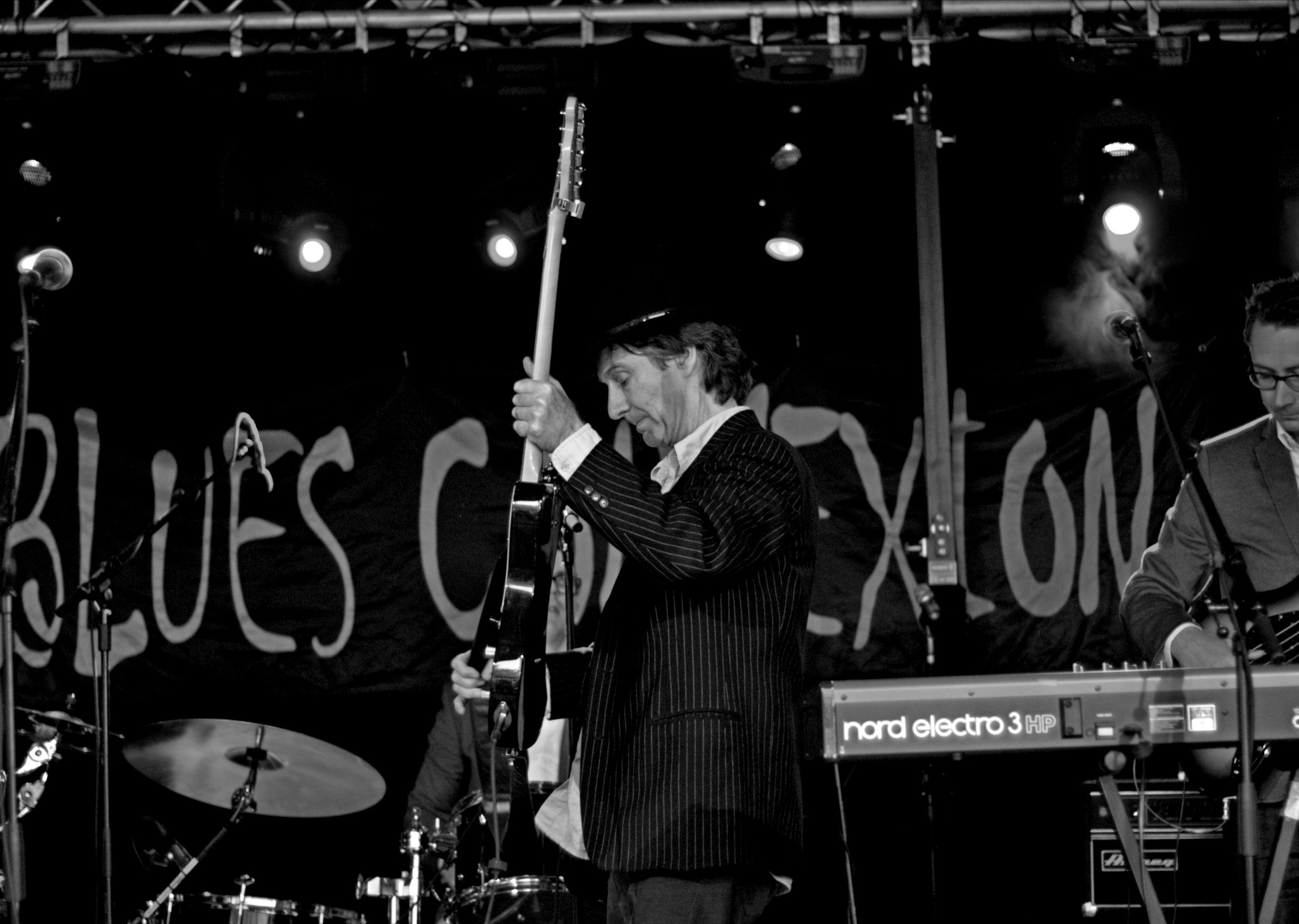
[120,718,385,819]
[14,704,125,739]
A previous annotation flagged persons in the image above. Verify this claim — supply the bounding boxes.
[403,303,815,924]
[1117,270,1299,924]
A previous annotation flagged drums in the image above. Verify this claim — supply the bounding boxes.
[453,788,574,911]
[191,890,367,924]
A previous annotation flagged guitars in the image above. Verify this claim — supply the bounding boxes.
[470,96,587,751]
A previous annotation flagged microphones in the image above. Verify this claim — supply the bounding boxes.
[20,247,72,291]
[1103,310,1140,346]
[242,413,274,493]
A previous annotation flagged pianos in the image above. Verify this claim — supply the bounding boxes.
[819,659,1299,924]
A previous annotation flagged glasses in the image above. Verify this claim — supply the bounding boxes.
[1244,363,1299,392]
